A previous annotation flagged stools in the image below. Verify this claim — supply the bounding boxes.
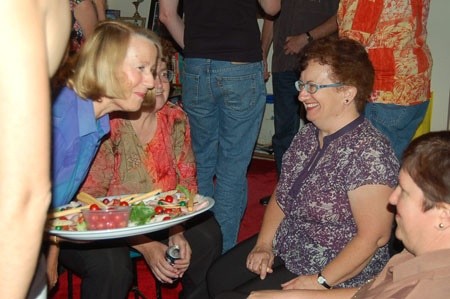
[67,248,161,299]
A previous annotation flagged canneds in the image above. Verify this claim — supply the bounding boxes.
[163,245,182,288]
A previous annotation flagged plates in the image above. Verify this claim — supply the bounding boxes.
[45,192,215,241]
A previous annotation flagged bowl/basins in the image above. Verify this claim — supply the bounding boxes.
[81,206,131,230]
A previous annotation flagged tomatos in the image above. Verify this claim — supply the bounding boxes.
[50,195,174,230]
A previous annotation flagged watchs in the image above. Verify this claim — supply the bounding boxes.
[305,31,313,42]
[317,270,333,289]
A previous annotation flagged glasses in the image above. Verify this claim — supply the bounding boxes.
[153,69,175,83]
[295,80,342,94]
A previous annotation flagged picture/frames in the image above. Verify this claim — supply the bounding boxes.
[148,1,183,38]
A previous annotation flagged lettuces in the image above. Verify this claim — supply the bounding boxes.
[128,183,190,226]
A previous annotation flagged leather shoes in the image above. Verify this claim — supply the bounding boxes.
[259,196,271,205]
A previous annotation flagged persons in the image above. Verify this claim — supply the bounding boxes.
[0,0,450,299]
